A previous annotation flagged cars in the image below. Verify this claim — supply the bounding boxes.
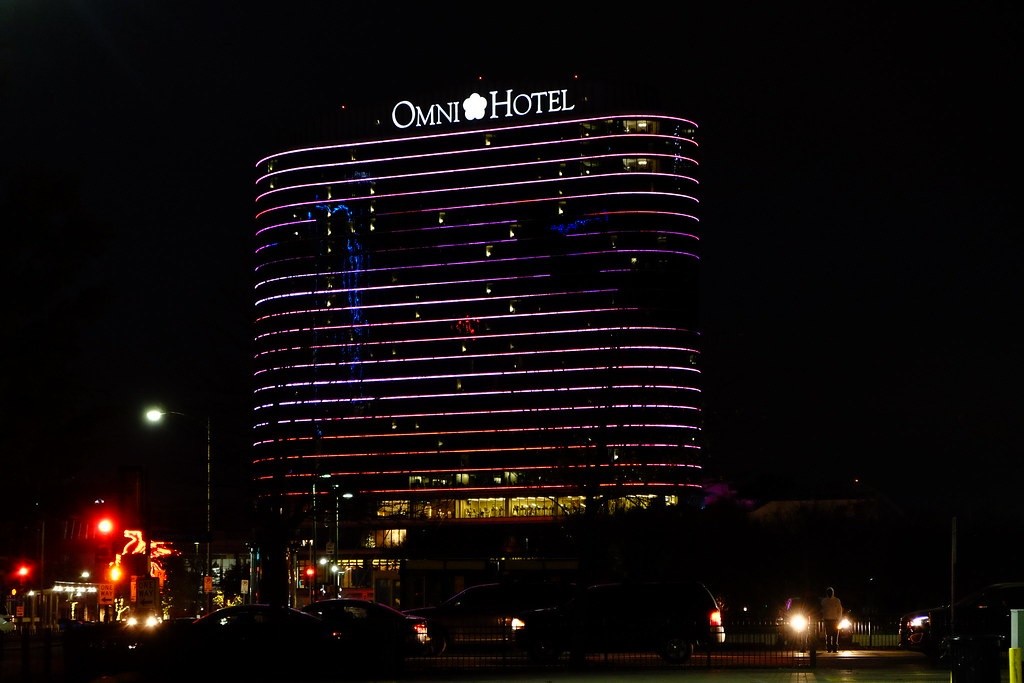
[510,580,725,665]
[60,580,539,683]
[774,595,856,654]
[898,580,1024,672]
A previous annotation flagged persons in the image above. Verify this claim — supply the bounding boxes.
[821,587,843,654]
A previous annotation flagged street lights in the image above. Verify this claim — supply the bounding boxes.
[310,472,355,601]
[146,407,214,615]
